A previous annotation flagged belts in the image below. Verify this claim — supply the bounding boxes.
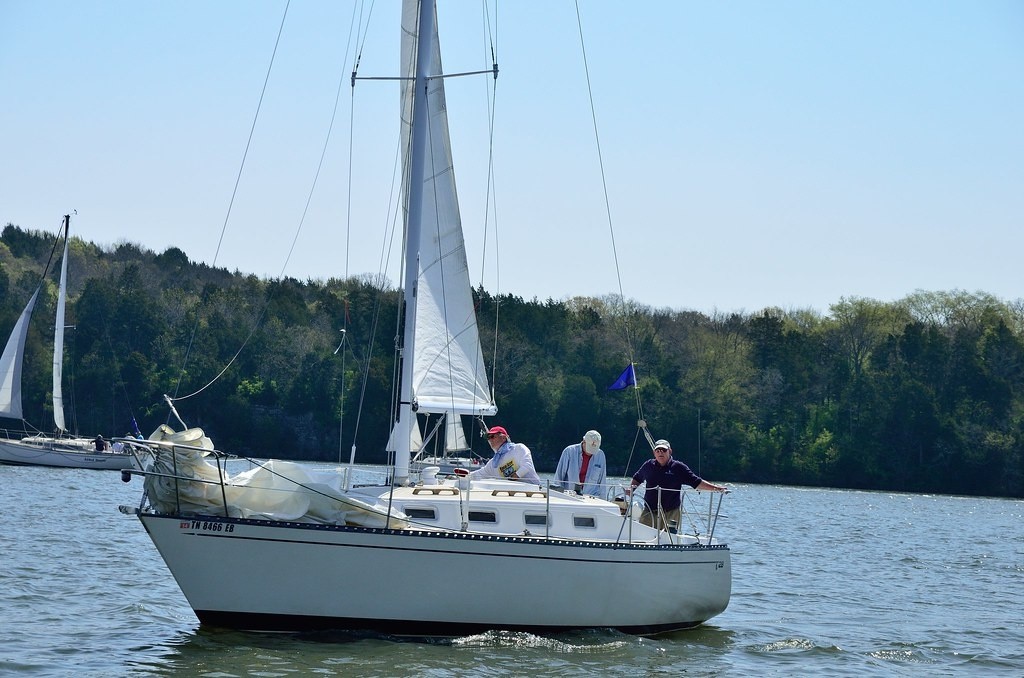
[646,508,672,514]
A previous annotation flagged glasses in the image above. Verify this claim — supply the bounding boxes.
[656,449,667,453]
[486,435,494,440]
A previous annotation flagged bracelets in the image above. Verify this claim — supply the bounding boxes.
[714,486,717,491]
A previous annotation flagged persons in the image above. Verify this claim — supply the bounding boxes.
[554,430,606,500]
[112,440,124,452]
[124,432,137,451]
[90,435,111,452]
[136,430,144,451]
[465,426,541,487]
[625,440,728,534]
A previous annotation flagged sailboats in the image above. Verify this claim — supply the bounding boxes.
[96,0,730,643]
[0,213,154,469]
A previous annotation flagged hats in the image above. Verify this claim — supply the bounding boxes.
[654,439,670,450]
[483,426,507,439]
[584,430,601,454]
